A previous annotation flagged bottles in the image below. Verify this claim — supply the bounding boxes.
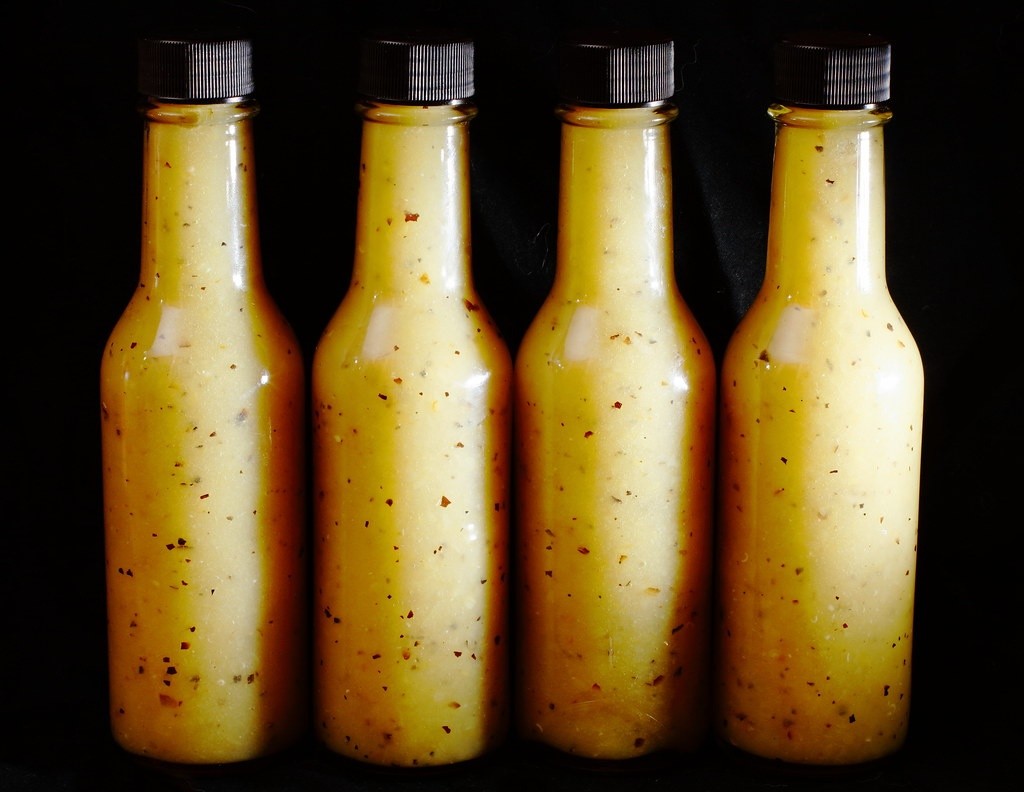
[720,35,928,765]
[512,36,720,759]
[310,35,514,765]
[100,41,303,765]
